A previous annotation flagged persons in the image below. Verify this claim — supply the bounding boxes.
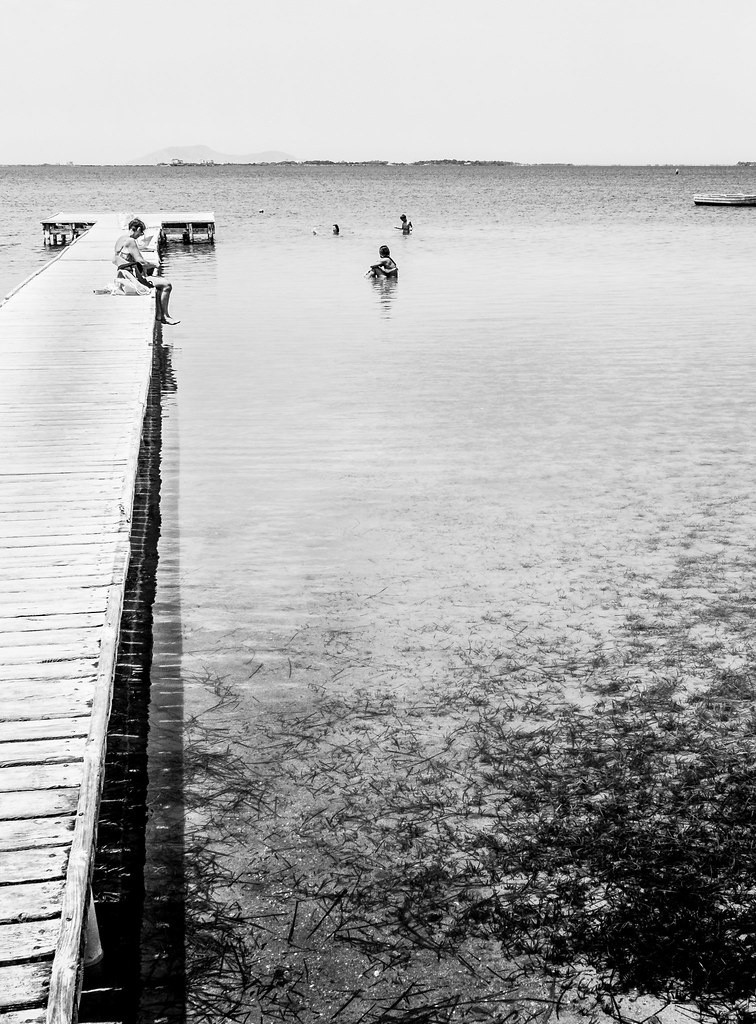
[312,223,340,234]
[365,245,398,278]
[395,214,413,234]
[113,218,180,325]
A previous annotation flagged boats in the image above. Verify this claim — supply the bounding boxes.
[693,193,756,208]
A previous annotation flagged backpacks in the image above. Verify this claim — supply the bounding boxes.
[117,262,154,291]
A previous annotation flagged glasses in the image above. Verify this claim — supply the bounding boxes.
[141,231,144,235]
